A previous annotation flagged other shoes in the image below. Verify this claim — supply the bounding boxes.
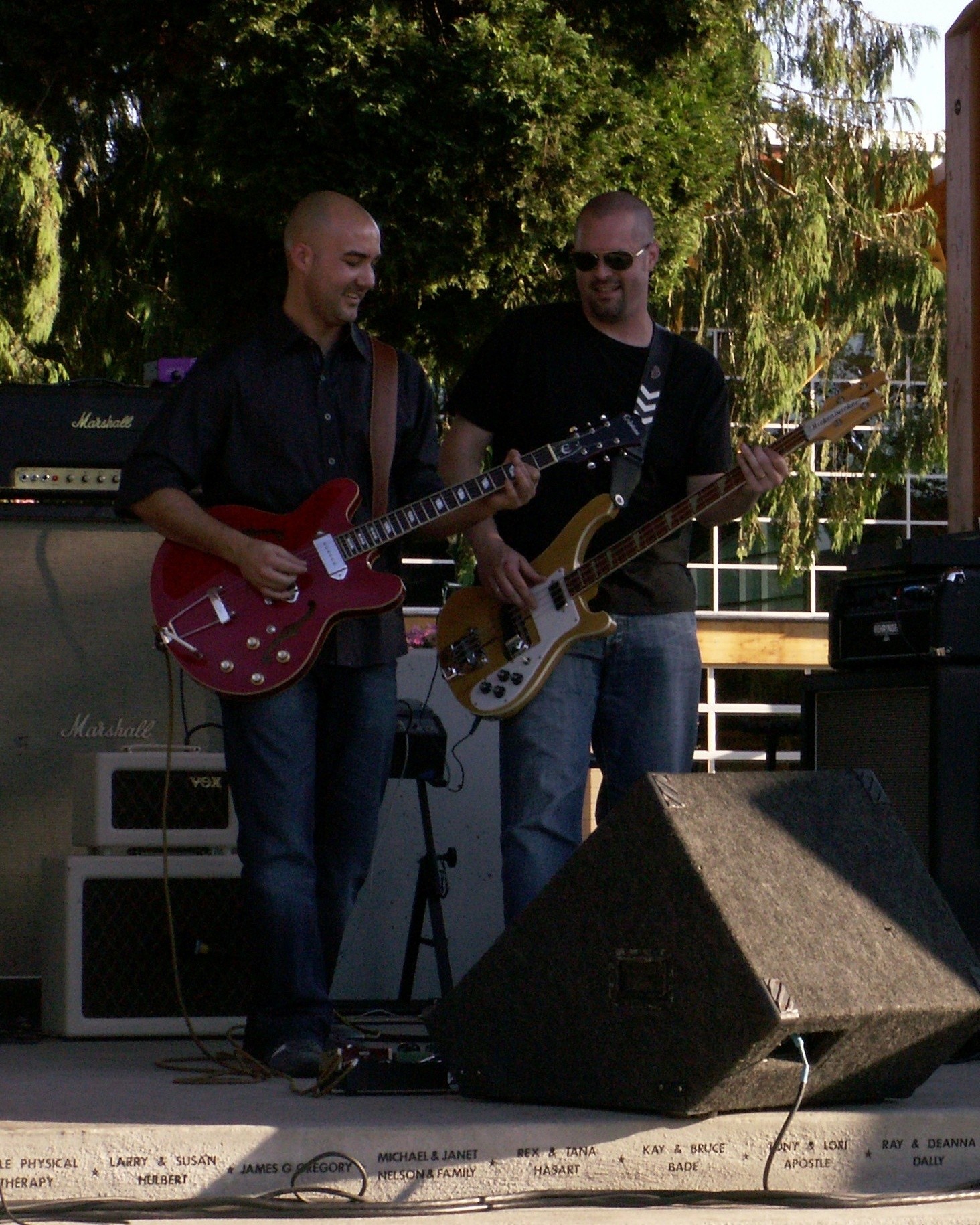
[290,1028,363,1056]
[239,1036,325,1079]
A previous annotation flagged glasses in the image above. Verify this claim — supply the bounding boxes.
[571,243,648,272]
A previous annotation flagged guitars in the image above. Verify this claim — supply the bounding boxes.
[436,367,889,720]
[151,413,649,699]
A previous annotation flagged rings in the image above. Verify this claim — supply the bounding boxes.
[757,473,766,481]
[489,587,500,595]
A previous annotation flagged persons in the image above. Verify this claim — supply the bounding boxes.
[115,190,541,1079]
[438,191,791,928]
